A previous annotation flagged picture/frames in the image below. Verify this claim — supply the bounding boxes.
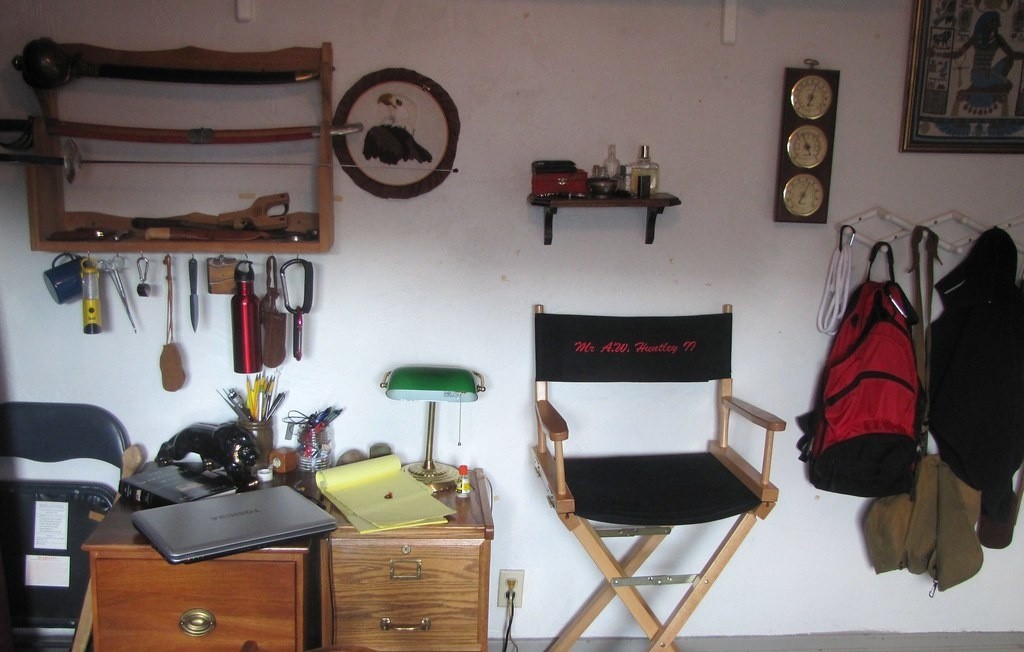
[898,0,1024,155]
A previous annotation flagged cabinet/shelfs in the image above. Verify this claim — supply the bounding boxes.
[81,462,317,652]
[319,468,495,652]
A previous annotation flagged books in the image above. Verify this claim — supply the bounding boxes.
[118,465,238,508]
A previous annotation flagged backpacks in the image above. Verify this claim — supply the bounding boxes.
[801,243,922,499]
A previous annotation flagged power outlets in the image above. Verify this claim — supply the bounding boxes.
[497,569,524,608]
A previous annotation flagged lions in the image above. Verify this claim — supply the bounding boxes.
[155,423,260,487]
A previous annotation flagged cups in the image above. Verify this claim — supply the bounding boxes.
[637,175,651,199]
[43,253,85,303]
[237,418,275,469]
[296,427,334,471]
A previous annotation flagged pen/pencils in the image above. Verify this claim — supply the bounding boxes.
[298,407,343,444]
[254,369,286,422]
[247,375,255,419]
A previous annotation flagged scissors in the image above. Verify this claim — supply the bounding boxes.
[98,256,137,333]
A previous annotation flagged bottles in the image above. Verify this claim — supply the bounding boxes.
[604,145,620,179]
[455,465,471,498]
[591,164,623,192]
[630,145,660,196]
[80,258,103,334]
[231,261,263,373]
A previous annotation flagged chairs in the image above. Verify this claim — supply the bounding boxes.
[0,401,131,652]
[531,303,785,652]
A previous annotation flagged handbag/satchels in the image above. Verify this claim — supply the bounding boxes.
[859,453,983,593]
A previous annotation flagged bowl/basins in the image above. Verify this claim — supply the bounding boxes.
[589,178,619,197]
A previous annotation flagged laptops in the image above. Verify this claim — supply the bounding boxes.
[129,485,339,565]
